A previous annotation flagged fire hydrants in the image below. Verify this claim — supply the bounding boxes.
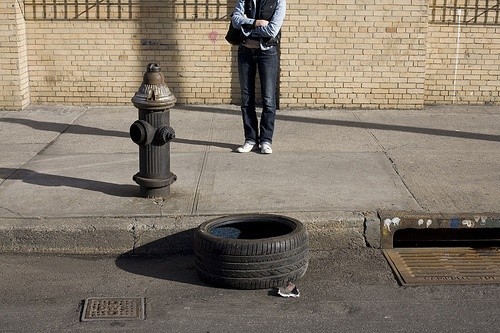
[130,62,177,198]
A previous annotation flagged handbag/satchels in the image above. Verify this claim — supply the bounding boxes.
[225,0,252,45]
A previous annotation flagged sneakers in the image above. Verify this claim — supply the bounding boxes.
[259,143,273,154]
[236,143,260,153]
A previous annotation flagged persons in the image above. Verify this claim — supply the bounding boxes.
[231,0,286,153]
